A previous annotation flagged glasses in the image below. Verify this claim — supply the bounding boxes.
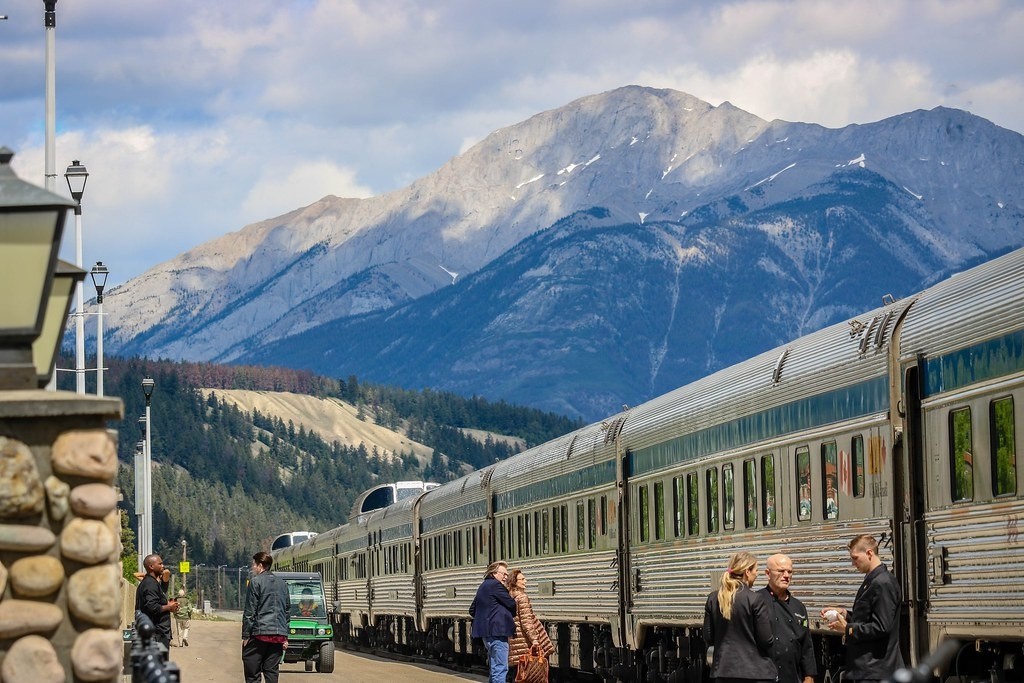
[517,578,525,580]
[497,571,508,576]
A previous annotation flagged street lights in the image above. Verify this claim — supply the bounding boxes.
[196,564,206,610]
[63,161,90,395]
[182,539,187,593]
[90,262,107,402]
[239,566,248,610]
[218,565,227,609]
[133,378,154,582]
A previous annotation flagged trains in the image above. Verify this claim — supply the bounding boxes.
[271,250,1024,683]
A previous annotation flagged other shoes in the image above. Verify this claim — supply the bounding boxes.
[183,638,188,646]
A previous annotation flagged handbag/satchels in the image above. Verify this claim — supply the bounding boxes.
[515,643,549,683]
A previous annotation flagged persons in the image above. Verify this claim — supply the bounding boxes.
[241,551,291,683]
[135,554,181,661]
[702,551,779,683]
[291,587,324,617]
[133,570,170,598]
[468,560,518,683]
[172,589,193,647]
[506,568,555,683]
[755,554,818,683]
[819,534,906,683]
